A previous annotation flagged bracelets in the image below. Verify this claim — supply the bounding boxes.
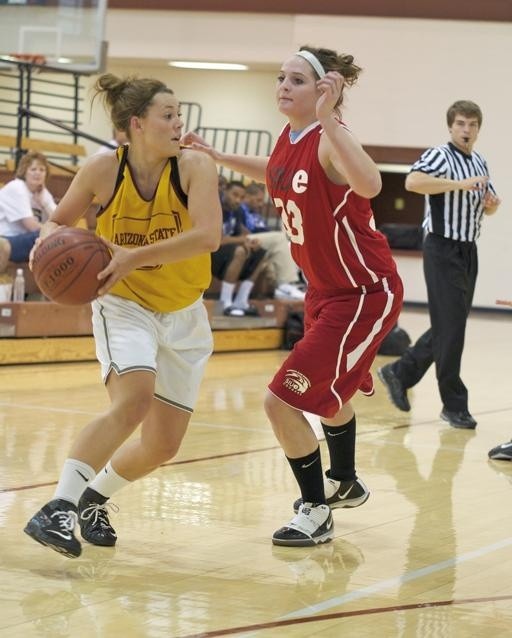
[40,196,53,209]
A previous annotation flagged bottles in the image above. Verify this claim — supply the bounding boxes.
[12,269,25,302]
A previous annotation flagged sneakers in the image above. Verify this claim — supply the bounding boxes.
[224,305,258,316]
[272,470,370,546]
[360,363,410,411]
[489,442,512,459]
[441,406,476,428]
[24,499,119,557]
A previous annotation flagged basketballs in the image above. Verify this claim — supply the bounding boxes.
[31,225,111,304]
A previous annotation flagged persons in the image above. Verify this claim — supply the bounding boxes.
[377,98,501,429]
[24,72,224,559]
[211,182,267,318]
[216,174,228,202]
[382,425,475,638]
[177,45,404,547]
[0,151,57,263]
[238,184,271,232]
[487,441,512,461]
[0,239,12,273]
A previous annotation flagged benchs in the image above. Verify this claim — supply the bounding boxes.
[0,161,302,364]
